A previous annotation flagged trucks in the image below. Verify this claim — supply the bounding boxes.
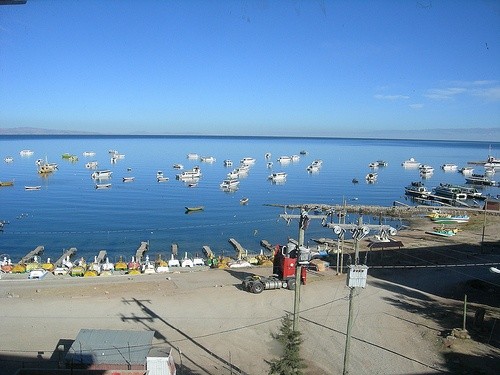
[240,242,311,294]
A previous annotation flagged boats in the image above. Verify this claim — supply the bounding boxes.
[184,206,203,211]
[19,149,34,155]
[364,156,500,202]
[122,176,135,180]
[490,266,500,274]
[62,150,127,189]
[424,207,470,238]
[264,149,324,184]
[352,177,359,184]
[239,197,249,204]
[4,157,13,163]
[0,248,206,280]
[24,185,42,189]
[0,182,12,186]
[35,154,59,174]
[156,149,258,193]
